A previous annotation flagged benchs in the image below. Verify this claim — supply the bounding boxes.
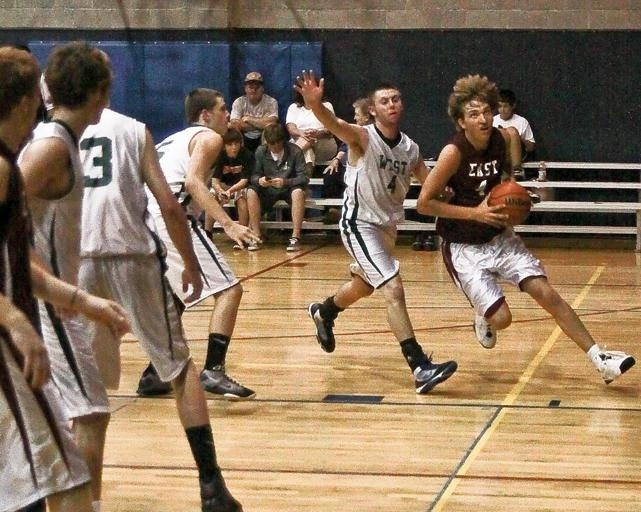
[201,158,640,251]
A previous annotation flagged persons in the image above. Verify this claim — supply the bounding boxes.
[230,73,374,250]
[422,74,634,385]
[492,90,536,176]
[295,71,458,393]
[2,41,262,512]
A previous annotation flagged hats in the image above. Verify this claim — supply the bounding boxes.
[243,71,264,83]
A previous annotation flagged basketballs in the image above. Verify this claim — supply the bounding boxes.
[488,182,532,226]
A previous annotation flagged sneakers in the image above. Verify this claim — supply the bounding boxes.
[323,207,340,225]
[308,302,336,353]
[198,367,257,400]
[198,469,243,512]
[412,231,422,250]
[413,352,458,393]
[137,364,174,396]
[422,232,434,251]
[592,343,635,385]
[471,313,497,348]
[233,236,301,251]
